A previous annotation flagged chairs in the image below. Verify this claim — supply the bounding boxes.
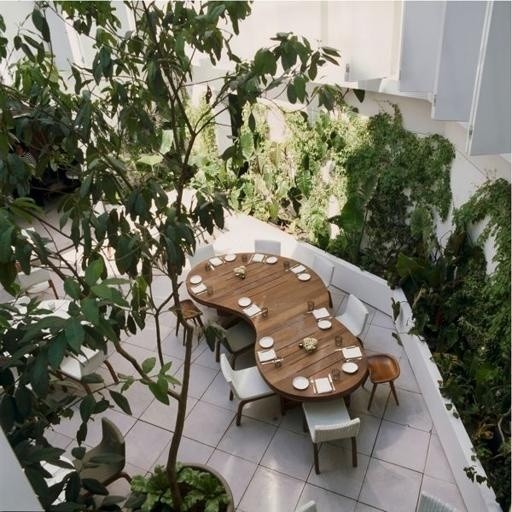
[72,417,132,501]
[1,226,119,395]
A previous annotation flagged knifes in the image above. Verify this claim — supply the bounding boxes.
[327,373,336,391]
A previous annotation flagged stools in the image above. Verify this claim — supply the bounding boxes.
[208,319,258,371]
[219,353,287,425]
[360,352,399,410]
[313,254,336,307]
[299,399,361,475]
[336,294,367,348]
[168,301,206,344]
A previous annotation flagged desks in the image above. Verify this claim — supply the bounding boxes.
[185,254,368,412]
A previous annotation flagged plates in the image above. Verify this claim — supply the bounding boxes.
[237,296,251,307]
[225,253,235,260]
[297,272,310,280]
[258,336,273,348]
[266,256,277,263]
[190,274,201,284]
[291,376,309,390]
[342,361,358,373]
[317,319,332,330]
